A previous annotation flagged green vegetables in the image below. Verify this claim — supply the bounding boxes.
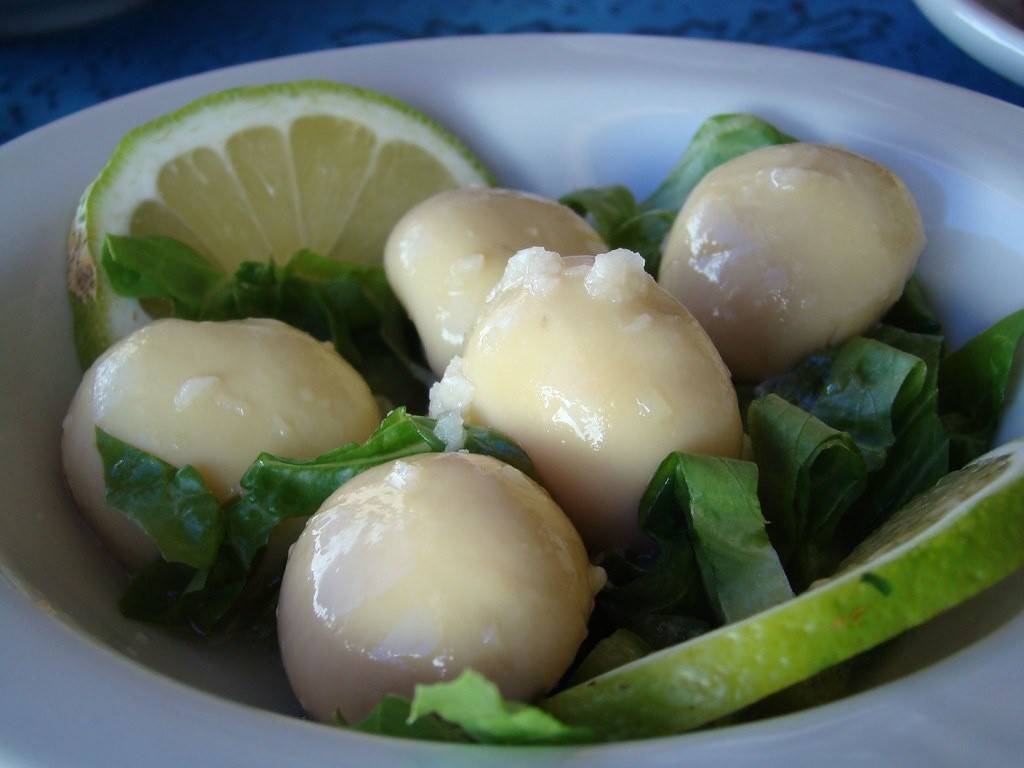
[99,109,1024,768]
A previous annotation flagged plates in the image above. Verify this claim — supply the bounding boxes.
[0,34,1024,768]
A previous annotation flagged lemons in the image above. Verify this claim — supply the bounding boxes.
[542,441,1023,739]
[68,79,496,382]
